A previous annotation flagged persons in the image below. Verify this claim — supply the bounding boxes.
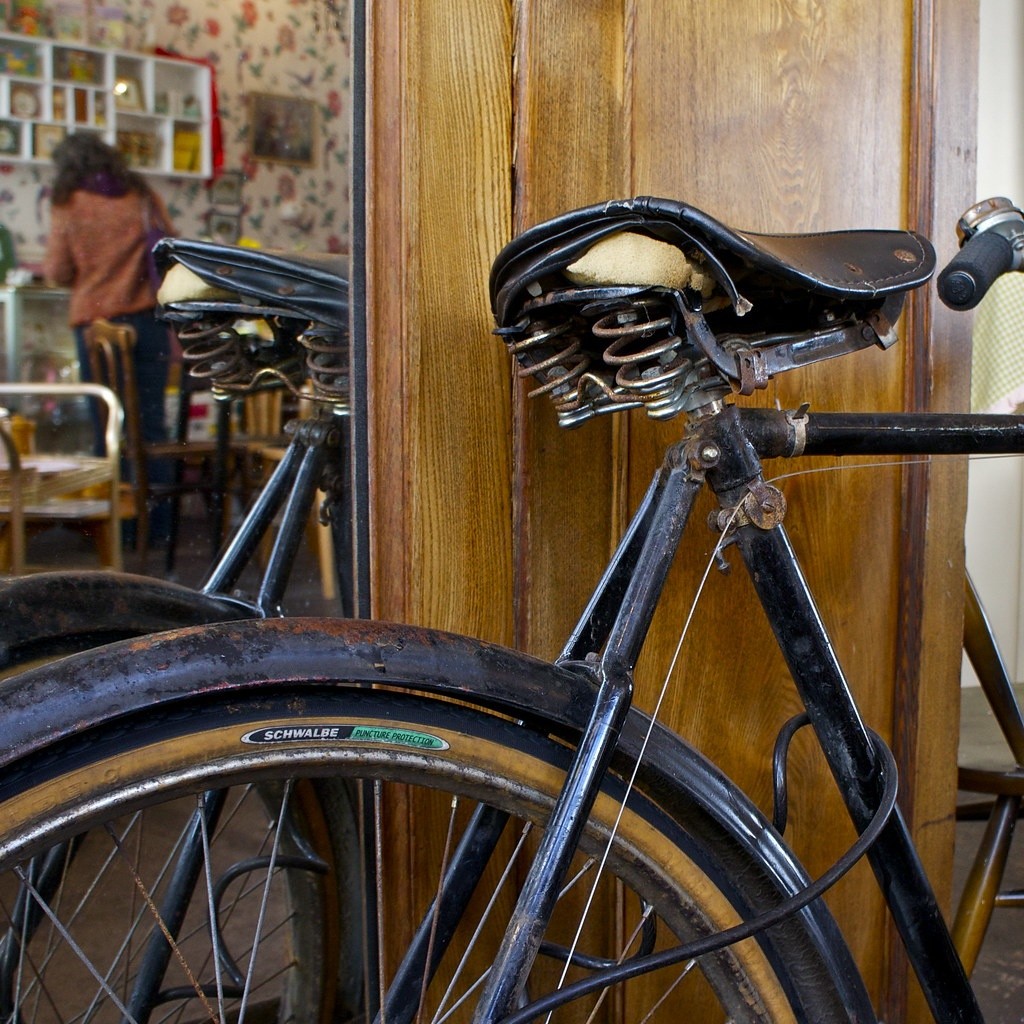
[45,132,176,553]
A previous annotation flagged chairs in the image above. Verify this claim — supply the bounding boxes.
[0,318,333,599]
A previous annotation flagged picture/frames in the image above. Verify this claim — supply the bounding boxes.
[248,92,317,167]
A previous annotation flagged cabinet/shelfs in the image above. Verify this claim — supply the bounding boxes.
[0,32,211,179]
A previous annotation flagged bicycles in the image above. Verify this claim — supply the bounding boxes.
[0,192,1021,1023]
[1,235,348,1023]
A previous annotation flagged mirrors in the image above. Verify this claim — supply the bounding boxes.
[0,0,375,1024]
